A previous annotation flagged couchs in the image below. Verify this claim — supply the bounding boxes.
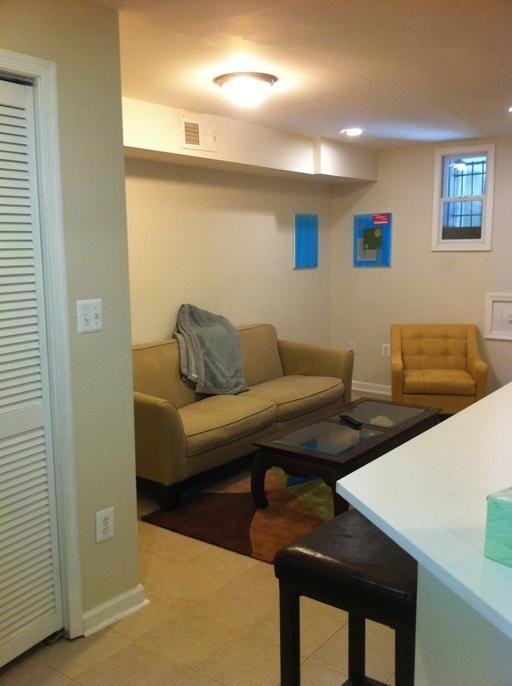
[134,322,354,506]
[390,324,488,415]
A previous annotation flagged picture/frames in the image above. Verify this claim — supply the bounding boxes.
[293,212,319,270]
[353,213,392,268]
[483,293,512,341]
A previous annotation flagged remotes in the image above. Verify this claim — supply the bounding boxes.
[340,411,363,428]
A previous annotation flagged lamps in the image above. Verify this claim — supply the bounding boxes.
[213,72,277,112]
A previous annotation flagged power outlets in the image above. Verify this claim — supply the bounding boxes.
[95,507,115,543]
[381,343,390,358]
[77,297,104,334]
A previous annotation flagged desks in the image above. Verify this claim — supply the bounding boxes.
[336,378,512,685]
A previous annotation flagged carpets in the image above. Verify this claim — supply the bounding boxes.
[140,458,355,565]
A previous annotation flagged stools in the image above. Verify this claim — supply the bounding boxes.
[274,510,417,686]
